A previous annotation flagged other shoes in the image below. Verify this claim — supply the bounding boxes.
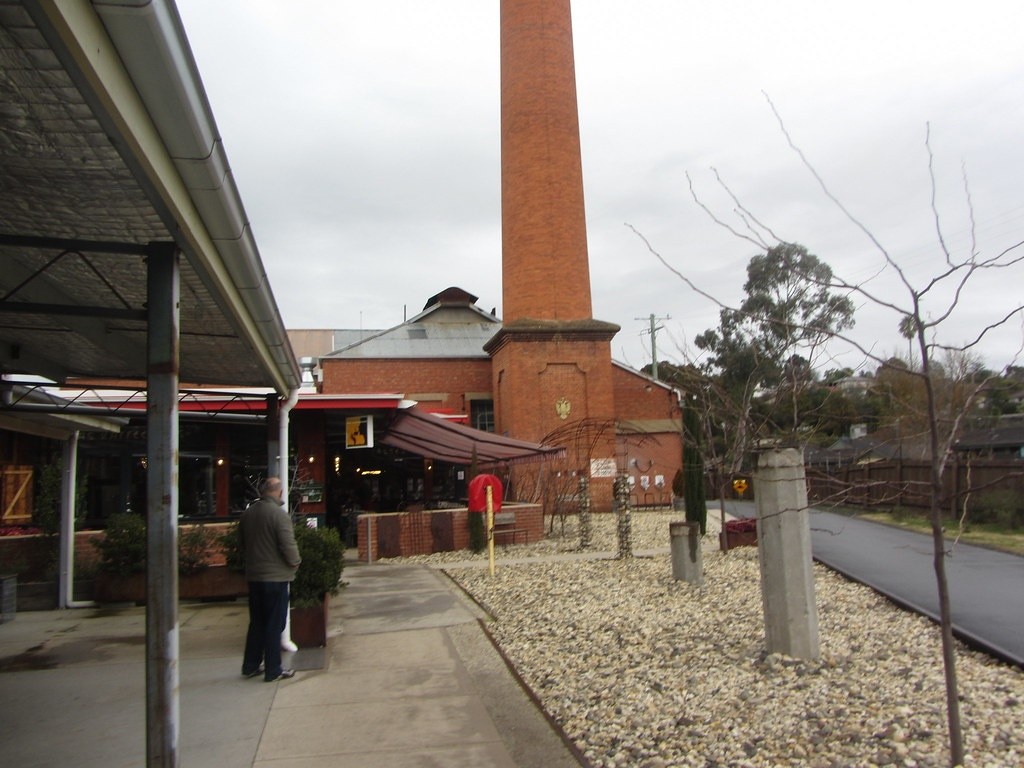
[242,660,265,678]
[264,668,296,682]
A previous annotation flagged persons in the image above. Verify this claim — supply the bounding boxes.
[236,477,302,682]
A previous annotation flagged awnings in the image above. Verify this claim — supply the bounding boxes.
[379,399,568,472]
